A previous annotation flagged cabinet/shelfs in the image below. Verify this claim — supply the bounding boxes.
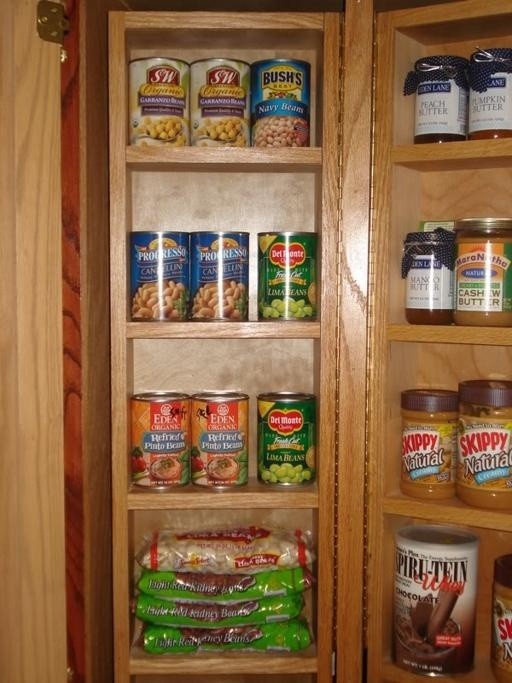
[365,0,512,683]
[108,9,337,682]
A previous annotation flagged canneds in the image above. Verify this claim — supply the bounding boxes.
[128,230,250,320]
[130,391,248,491]
[401,379,511,508]
[128,57,310,149]
[400,227,455,323]
[258,232,317,319]
[256,392,316,487]
[414,56,468,141]
[467,46,511,140]
[455,218,511,326]
[393,524,512,680]
